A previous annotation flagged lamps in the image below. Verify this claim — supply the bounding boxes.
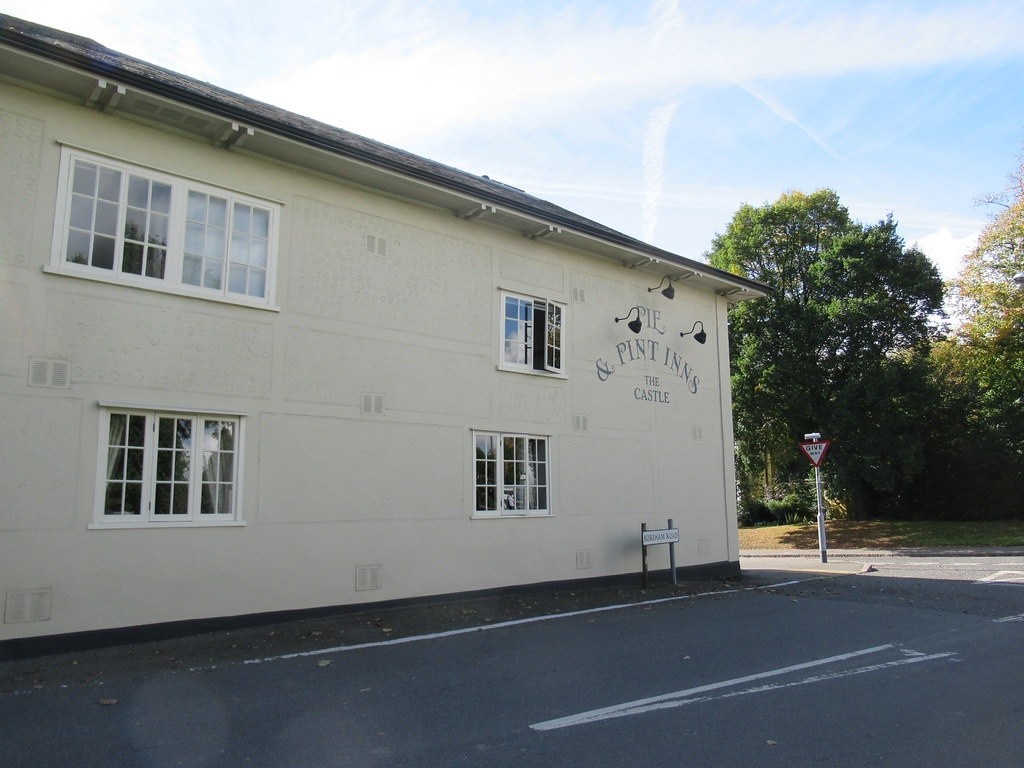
[648,276,675,299]
[615,307,642,334]
[680,321,706,344]
